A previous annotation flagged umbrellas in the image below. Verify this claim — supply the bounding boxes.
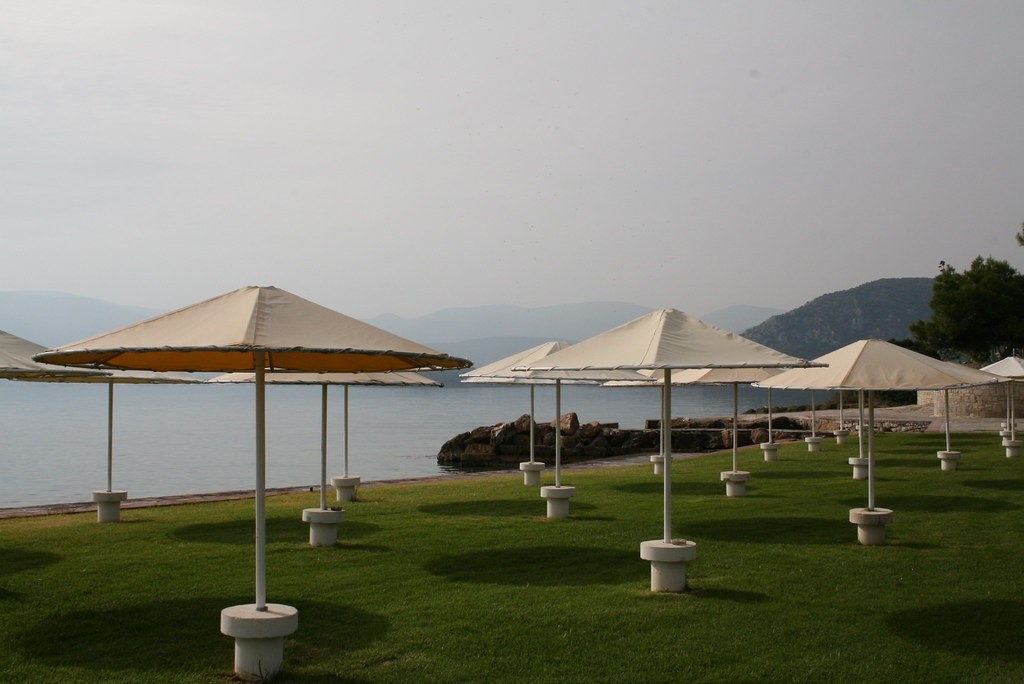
[0,284,1024,610]
